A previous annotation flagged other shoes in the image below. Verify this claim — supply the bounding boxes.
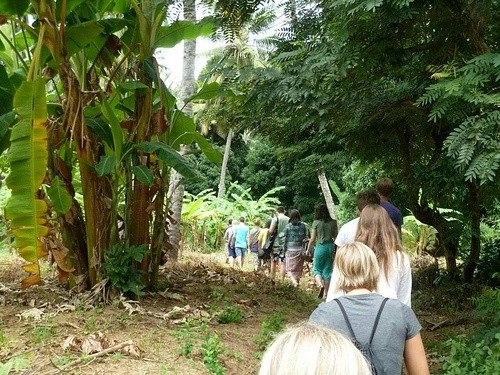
[317,286,325,299]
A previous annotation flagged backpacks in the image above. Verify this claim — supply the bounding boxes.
[335,297,389,375]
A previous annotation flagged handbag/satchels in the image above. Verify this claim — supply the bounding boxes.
[302,223,312,262]
[258,250,271,259]
[262,232,277,252]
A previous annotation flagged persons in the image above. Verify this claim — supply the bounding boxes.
[227,220,237,265]
[257,218,272,272]
[257,318,373,375]
[270,207,290,281]
[307,241,430,375]
[306,203,338,299]
[234,216,249,270]
[223,219,233,264]
[325,204,411,309]
[376,176,402,241]
[279,209,309,288]
[247,217,263,272]
[334,192,381,264]
